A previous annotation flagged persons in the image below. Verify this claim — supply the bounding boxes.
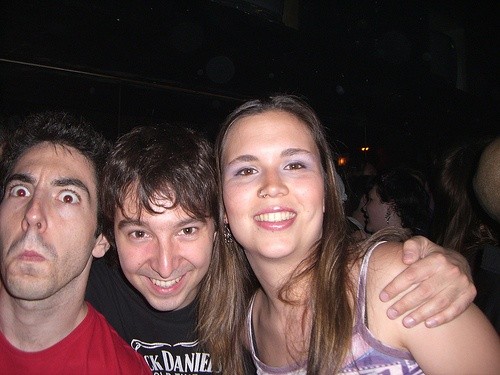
[360,170,434,239]
[198,93,500,375]
[85,122,480,374]
[422,139,499,335]
[347,149,390,231]
[1,110,153,375]
